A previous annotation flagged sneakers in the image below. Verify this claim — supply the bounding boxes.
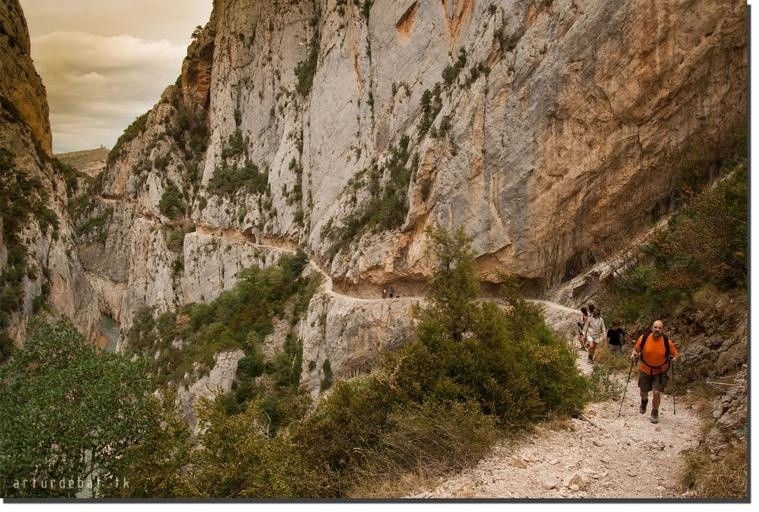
[588,354,594,364]
[651,409,658,423]
[640,399,648,413]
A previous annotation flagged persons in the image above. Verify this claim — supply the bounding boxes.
[581,308,607,364]
[382,288,407,299]
[587,305,594,316]
[578,306,588,352]
[631,320,679,423]
[605,318,626,355]
